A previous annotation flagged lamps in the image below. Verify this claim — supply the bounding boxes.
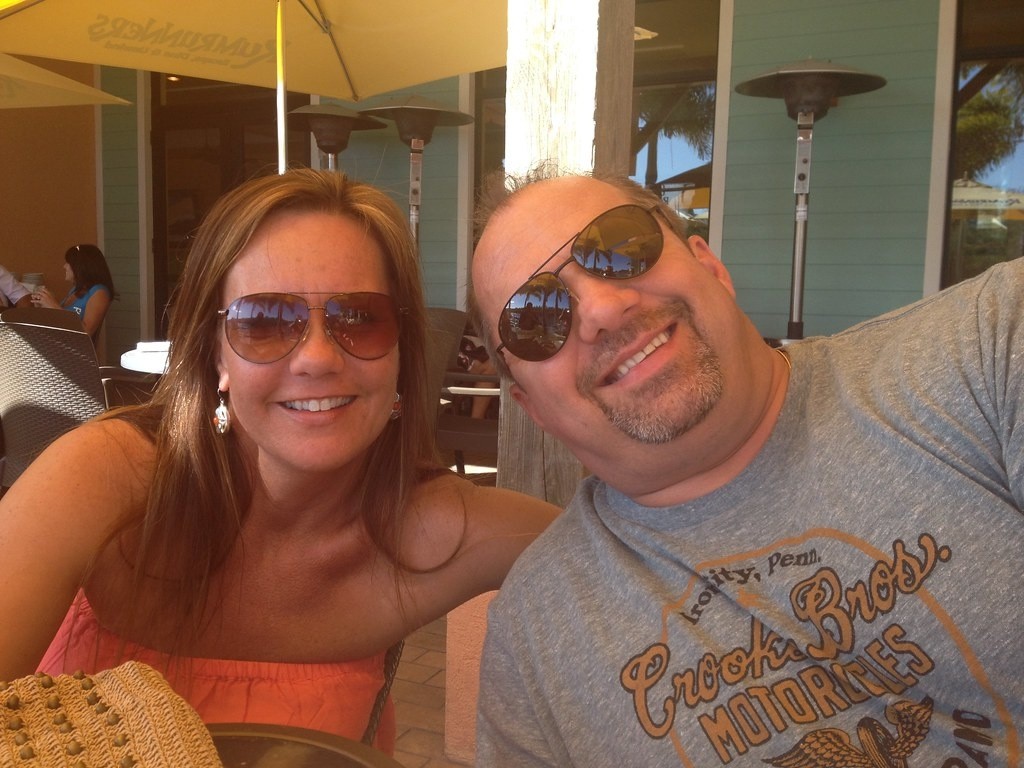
[288,96,475,268]
[733,55,887,348]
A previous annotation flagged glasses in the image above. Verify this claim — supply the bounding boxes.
[495,204,673,375]
[218,291,409,364]
[72,244,81,253]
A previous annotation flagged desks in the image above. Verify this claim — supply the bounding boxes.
[121,349,172,375]
[446,386,501,397]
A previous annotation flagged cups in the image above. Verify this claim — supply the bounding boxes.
[33,285,45,308]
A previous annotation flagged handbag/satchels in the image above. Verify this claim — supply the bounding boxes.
[0,660,224,768]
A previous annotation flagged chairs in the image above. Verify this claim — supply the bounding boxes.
[419,308,467,471]
[100,363,161,411]
[0,307,109,495]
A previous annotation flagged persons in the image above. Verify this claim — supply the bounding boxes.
[465,169,1024,768]
[0,163,562,768]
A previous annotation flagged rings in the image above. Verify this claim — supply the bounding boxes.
[37,295,40,299]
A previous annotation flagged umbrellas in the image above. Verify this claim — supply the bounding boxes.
[0,0,658,176]
